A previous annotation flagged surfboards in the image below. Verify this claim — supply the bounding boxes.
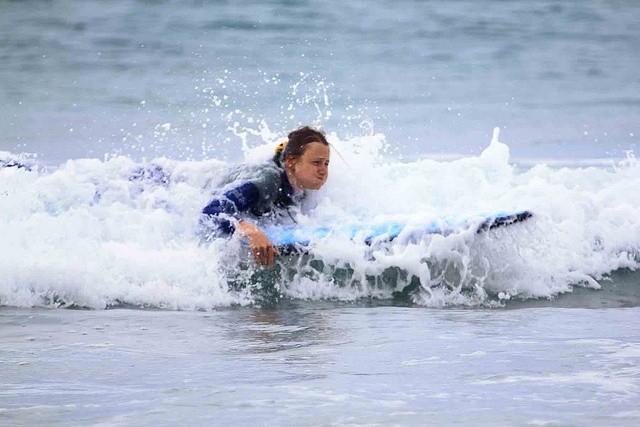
[235,209,533,256]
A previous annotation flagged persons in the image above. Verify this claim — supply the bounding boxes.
[198,125,331,267]
